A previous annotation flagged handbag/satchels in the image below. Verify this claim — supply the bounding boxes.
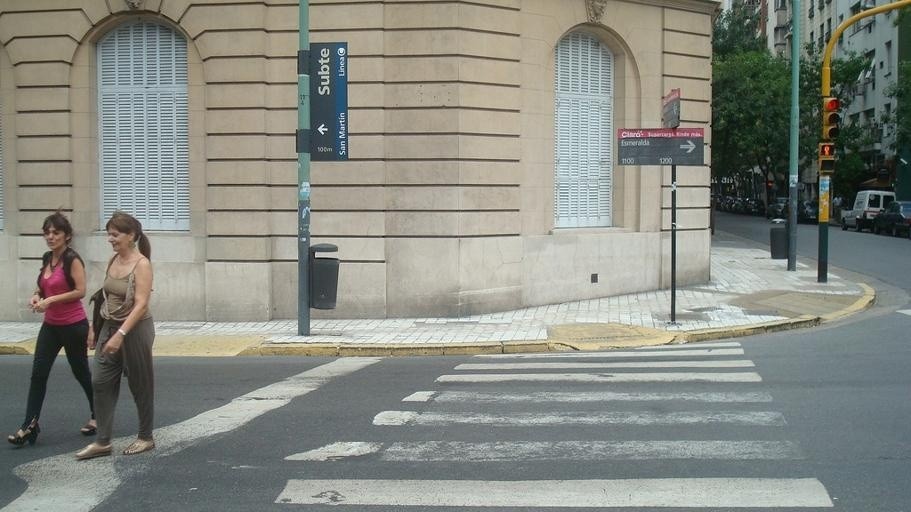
[87,286,104,344]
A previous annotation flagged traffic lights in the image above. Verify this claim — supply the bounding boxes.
[822,97,840,140]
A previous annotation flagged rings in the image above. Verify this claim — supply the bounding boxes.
[109,351,115,354]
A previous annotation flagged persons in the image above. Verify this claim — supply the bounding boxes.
[7,205,97,447]
[591,0,606,23]
[75,209,155,459]
[832,193,843,221]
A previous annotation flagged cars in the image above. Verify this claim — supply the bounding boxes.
[767,196,818,224]
[871,200,911,238]
[711,193,765,216]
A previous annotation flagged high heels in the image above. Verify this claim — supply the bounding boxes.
[81,421,96,435]
[8,423,41,446]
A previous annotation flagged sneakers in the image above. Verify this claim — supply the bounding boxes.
[123,439,155,456]
[76,442,112,459]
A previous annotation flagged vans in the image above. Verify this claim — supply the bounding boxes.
[841,190,897,232]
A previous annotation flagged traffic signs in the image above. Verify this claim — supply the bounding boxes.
[660,88,680,129]
[617,128,705,166]
[308,41,349,162]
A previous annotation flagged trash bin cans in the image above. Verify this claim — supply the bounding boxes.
[769,218,789,261]
[308,242,341,312]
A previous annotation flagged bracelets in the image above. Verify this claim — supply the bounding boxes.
[34,291,40,295]
[118,329,126,335]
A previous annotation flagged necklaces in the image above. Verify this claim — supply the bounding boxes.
[50,256,62,272]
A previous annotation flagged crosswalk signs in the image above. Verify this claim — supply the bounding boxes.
[818,142,836,157]
